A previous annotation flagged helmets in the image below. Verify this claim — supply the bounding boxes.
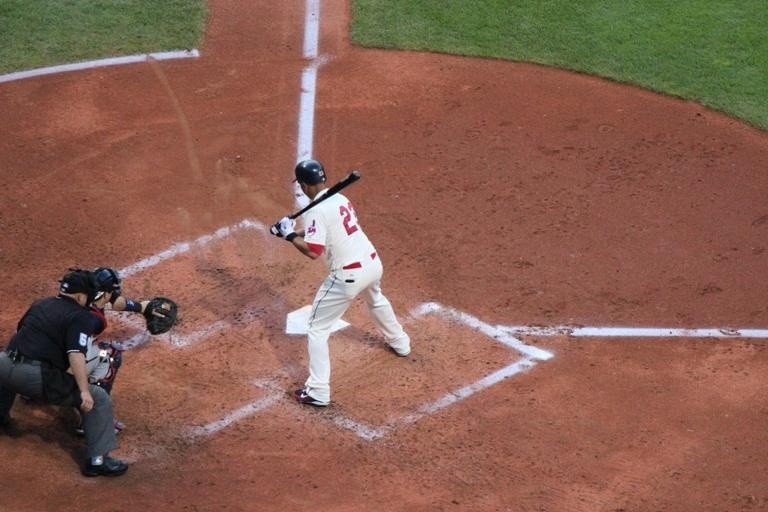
[91,266,122,305]
[292,159,326,185]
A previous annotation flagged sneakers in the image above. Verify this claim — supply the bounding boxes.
[292,388,330,406]
[82,457,129,478]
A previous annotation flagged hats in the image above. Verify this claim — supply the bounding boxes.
[59,270,91,294]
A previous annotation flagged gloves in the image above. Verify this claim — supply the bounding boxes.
[269,216,298,242]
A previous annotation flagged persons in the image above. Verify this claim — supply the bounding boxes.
[268,159,413,408]
[15,266,180,436]
[0,264,131,476]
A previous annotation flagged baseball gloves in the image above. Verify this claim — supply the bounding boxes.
[143,296,178,335]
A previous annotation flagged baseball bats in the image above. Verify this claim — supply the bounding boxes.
[271,168,362,237]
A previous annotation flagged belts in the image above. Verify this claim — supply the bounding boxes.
[6,348,33,366]
[344,252,376,269]
